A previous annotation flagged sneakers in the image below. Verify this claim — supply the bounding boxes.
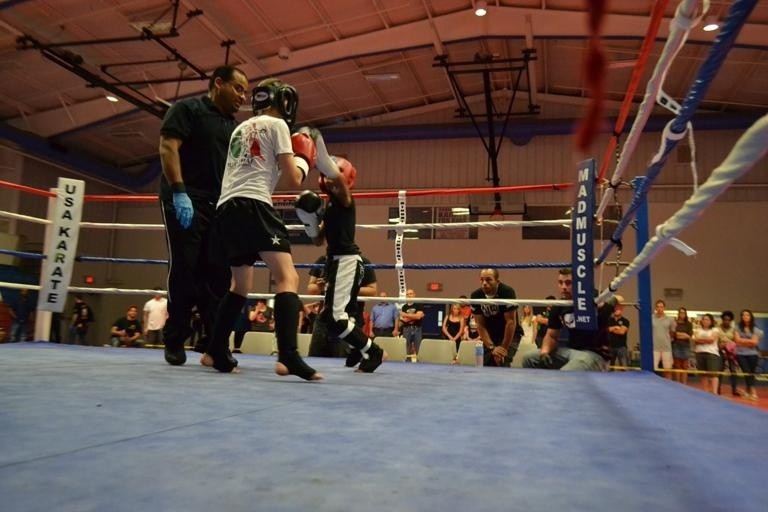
[164,341,187,366]
[194,339,238,367]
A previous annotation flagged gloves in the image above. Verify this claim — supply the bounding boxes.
[296,190,325,239]
[289,133,318,183]
[171,191,194,230]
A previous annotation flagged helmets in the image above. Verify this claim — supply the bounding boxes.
[317,155,359,197]
[250,77,301,130]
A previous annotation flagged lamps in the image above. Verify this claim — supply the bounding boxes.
[702,15,719,32]
[105,89,118,103]
[474,1,488,18]
[177,61,188,71]
[490,210,504,221]
[278,46,290,59]
[141,30,151,41]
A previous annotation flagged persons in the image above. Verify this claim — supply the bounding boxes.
[692,314,719,395]
[718,311,741,397]
[458,295,470,324]
[295,128,388,373]
[110,305,143,347]
[734,309,761,399]
[607,306,629,371]
[672,307,692,384]
[536,296,556,327]
[471,268,525,369]
[158,65,249,368]
[400,289,424,354]
[200,78,324,380]
[465,315,481,341]
[510,305,537,367]
[72,294,95,345]
[303,301,320,324]
[8,289,35,343]
[652,299,672,380]
[296,298,305,334]
[442,305,464,352]
[249,300,272,332]
[522,268,606,372]
[142,286,169,344]
[369,292,399,339]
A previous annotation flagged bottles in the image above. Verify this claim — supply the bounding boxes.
[474,340,484,368]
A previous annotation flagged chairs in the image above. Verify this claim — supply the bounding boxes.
[374,337,407,362]
[272,333,312,358]
[513,335,537,368]
[229,331,235,353]
[459,340,482,366]
[240,332,273,355]
[420,339,457,363]
